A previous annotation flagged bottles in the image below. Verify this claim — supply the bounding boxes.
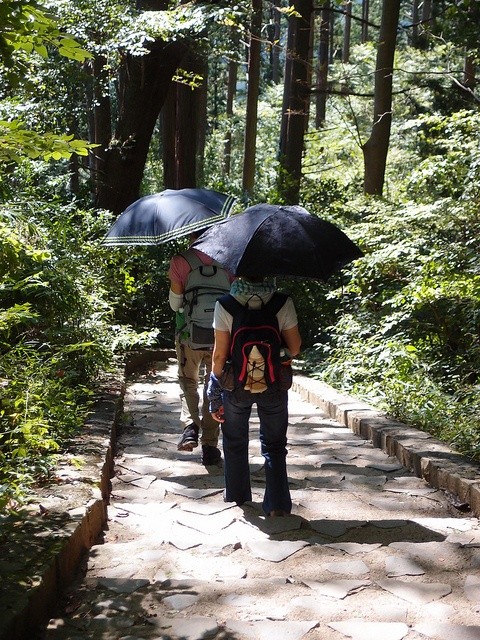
[176,307,185,330]
[279,347,287,364]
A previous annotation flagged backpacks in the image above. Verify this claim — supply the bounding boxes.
[177,249,230,352]
[215,292,292,401]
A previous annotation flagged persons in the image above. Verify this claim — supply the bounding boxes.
[207,275,302,516]
[168,227,238,465]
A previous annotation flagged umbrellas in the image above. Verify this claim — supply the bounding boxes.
[98,189,236,246]
[190,204,364,283]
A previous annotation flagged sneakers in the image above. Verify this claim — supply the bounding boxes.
[177,423,199,452]
[201,447,221,466]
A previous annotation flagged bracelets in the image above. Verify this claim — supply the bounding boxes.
[208,373,222,413]
